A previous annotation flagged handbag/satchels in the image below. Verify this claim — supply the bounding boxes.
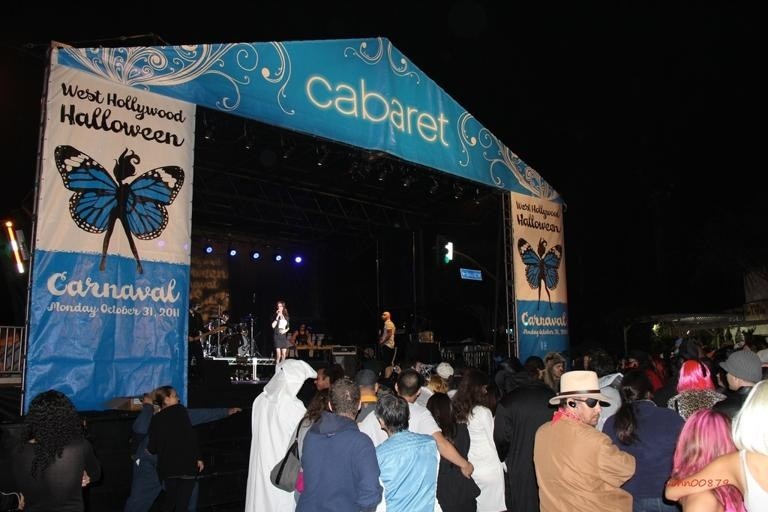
[269,414,310,493]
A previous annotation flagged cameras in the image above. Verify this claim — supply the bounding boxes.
[393,366,397,371]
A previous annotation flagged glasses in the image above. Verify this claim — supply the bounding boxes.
[574,397,598,407]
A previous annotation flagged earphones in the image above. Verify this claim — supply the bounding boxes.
[569,400,576,407]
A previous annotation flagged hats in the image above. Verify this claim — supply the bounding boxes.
[549,369,613,405]
[719,349,763,382]
[436,361,454,379]
[354,368,382,386]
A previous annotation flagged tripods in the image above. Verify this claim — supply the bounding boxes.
[242,318,262,356]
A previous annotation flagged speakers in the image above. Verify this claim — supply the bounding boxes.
[309,360,328,370]
[195,358,229,385]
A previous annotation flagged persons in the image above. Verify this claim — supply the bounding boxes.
[211,311,231,357]
[290,323,313,362]
[272,300,290,364]
[188,298,214,369]
[379,311,397,367]
[3,348,768,512]
